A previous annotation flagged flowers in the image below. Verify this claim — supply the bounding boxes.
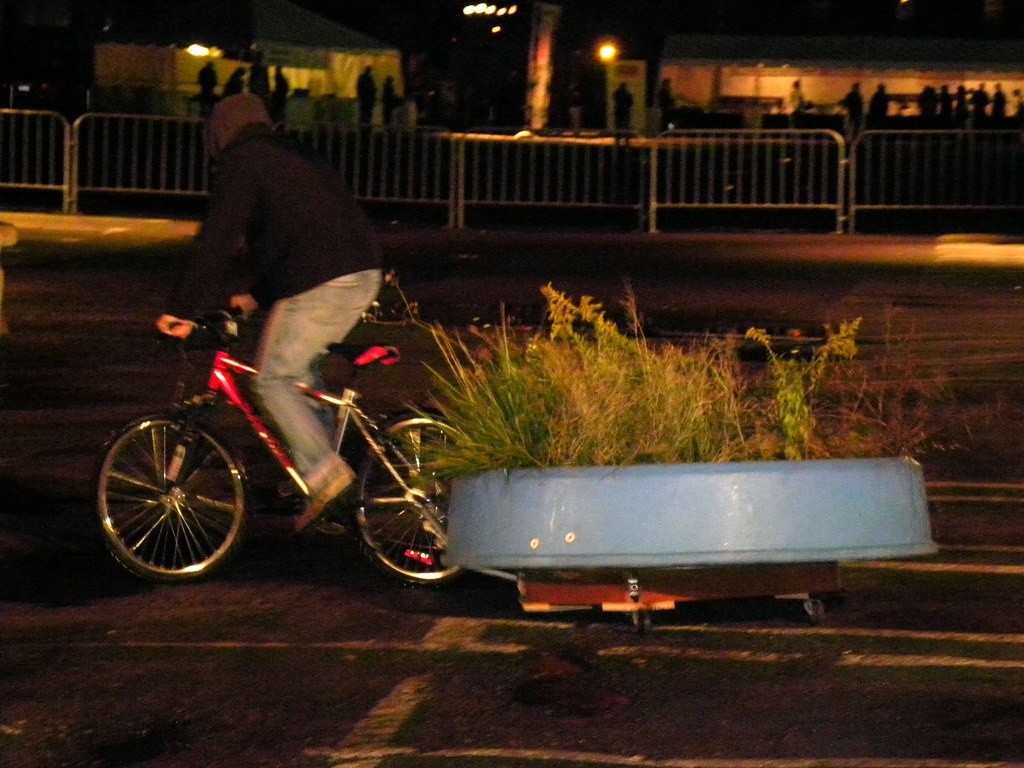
[361,267,997,489]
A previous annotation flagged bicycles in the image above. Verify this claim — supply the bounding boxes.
[91,310,490,588]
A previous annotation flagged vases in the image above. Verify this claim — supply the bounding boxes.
[443,456,937,613]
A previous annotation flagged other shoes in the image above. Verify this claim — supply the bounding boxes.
[294,466,363,538]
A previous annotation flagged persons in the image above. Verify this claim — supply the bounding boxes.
[956,85,970,118]
[937,84,954,118]
[845,83,862,133]
[612,82,632,136]
[869,84,889,130]
[918,86,936,115]
[657,79,674,109]
[159,91,382,531]
[275,66,289,96]
[1014,89,1024,118]
[198,61,217,93]
[383,77,395,123]
[250,62,271,94]
[224,66,244,95]
[791,80,802,109]
[972,84,989,118]
[992,84,1007,119]
[357,66,376,125]
